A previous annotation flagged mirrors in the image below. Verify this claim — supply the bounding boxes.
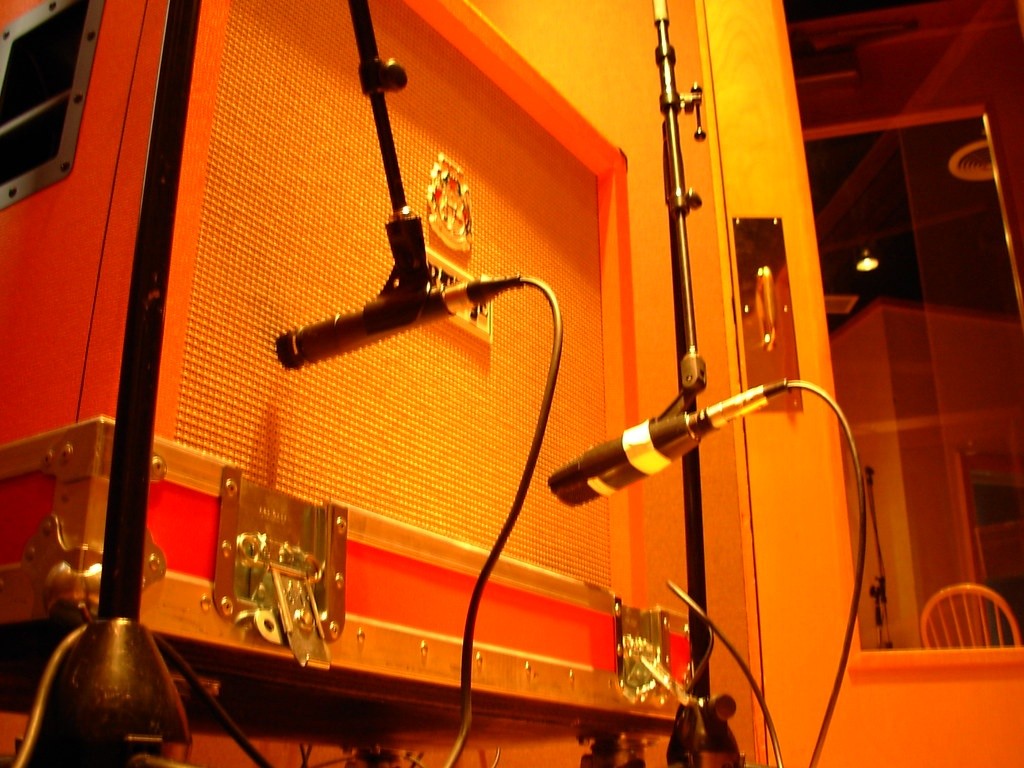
[801,104,1024,668]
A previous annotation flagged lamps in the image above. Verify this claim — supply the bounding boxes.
[855,247,879,272]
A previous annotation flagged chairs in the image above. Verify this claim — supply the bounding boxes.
[918,581,1023,648]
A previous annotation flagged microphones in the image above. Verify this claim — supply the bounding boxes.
[275,273,526,370]
[548,378,793,509]
